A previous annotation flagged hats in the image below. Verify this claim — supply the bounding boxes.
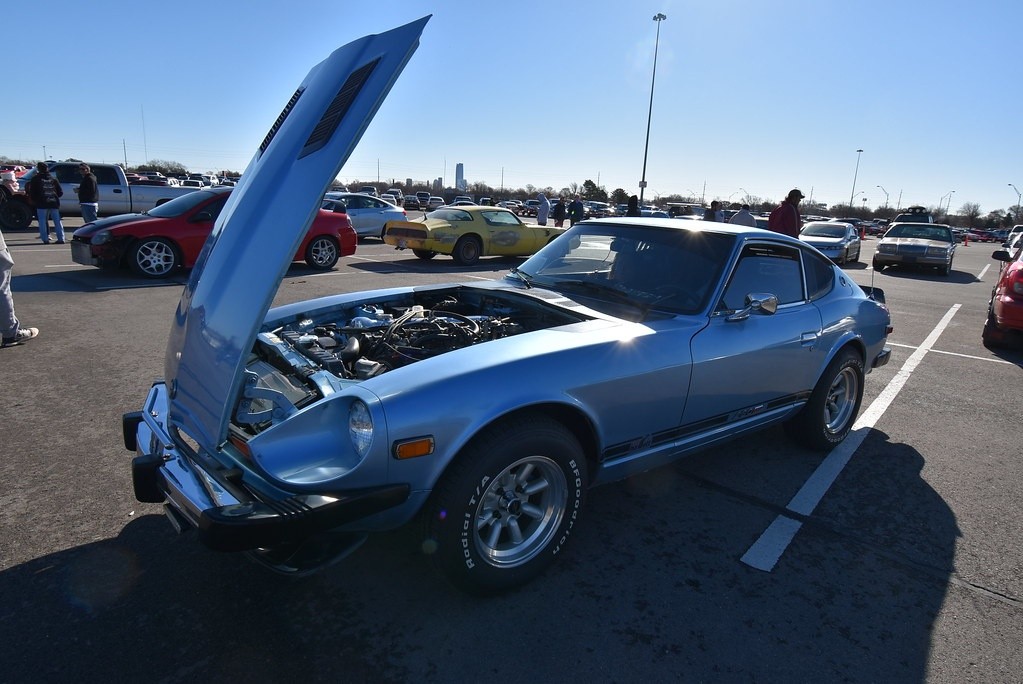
[790,190,805,198]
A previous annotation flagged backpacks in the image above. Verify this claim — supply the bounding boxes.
[37,177,59,204]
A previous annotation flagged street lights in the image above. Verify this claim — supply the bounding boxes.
[938,191,956,213]
[638,13,666,207]
[877,186,889,209]
[1008,184,1021,219]
[850,149,863,208]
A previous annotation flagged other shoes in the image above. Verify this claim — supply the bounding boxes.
[44,241,49,243]
[53,240,65,244]
[48,235,53,240]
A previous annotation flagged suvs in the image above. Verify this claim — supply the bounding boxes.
[888,206,933,231]
[454,196,494,206]
[523,198,574,216]
[378,194,446,211]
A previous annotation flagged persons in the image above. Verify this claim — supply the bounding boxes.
[768,189,805,238]
[489,198,499,206]
[626,195,641,216]
[536,193,550,226]
[498,198,506,208]
[728,204,757,227]
[568,195,583,227]
[74,163,99,222]
[552,197,566,227]
[0,179,39,346]
[30,162,66,244]
[671,205,694,218]
[704,201,724,222]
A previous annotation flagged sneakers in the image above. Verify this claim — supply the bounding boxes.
[2,328,39,346]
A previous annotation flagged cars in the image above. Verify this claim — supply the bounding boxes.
[70,187,358,279]
[325,192,409,242]
[125,171,240,189]
[583,202,770,230]
[382,200,577,265]
[122,9,895,600]
[797,213,1023,349]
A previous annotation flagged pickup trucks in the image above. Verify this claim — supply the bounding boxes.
[0,161,212,230]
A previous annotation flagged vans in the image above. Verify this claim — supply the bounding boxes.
[361,186,430,205]
